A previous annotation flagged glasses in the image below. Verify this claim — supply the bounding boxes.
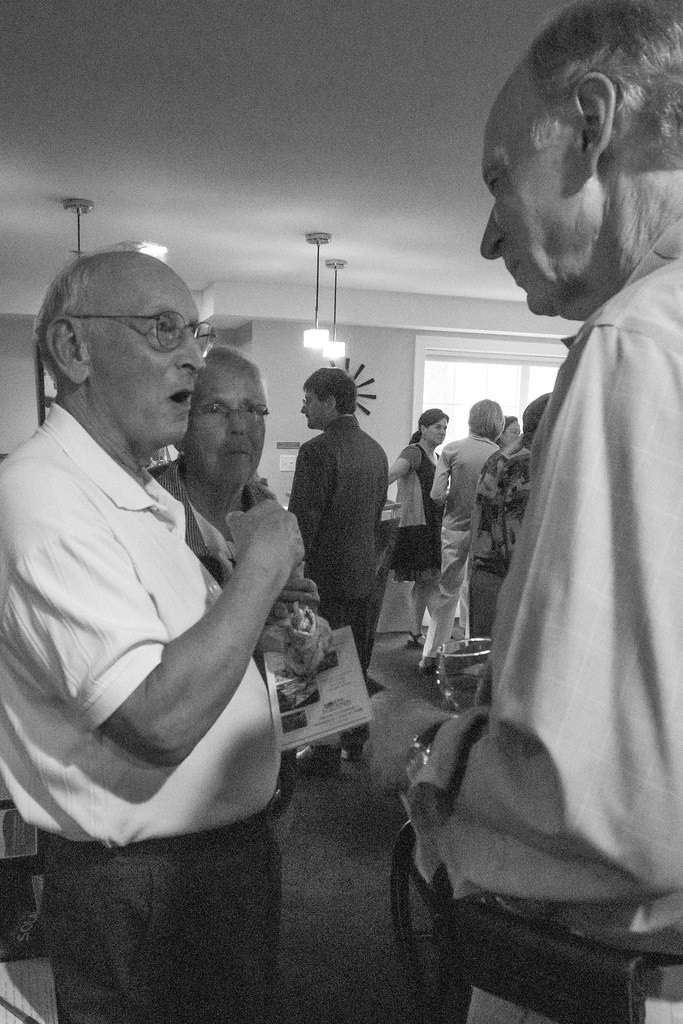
[302,398,327,405]
[190,403,269,426]
[64,311,215,358]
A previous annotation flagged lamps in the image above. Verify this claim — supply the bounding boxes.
[304,233,332,347]
[322,259,348,358]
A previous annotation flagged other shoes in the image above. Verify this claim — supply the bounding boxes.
[296,745,340,772]
[341,744,363,760]
[419,660,436,675]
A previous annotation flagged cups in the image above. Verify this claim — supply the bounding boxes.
[437,637,491,718]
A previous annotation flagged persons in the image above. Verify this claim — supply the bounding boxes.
[0,250,319,1024]
[389,0,683,1024]
[147,346,555,776]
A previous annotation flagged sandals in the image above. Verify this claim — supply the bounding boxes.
[406,631,426,647]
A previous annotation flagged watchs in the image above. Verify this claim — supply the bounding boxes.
[406,717,458,782]
